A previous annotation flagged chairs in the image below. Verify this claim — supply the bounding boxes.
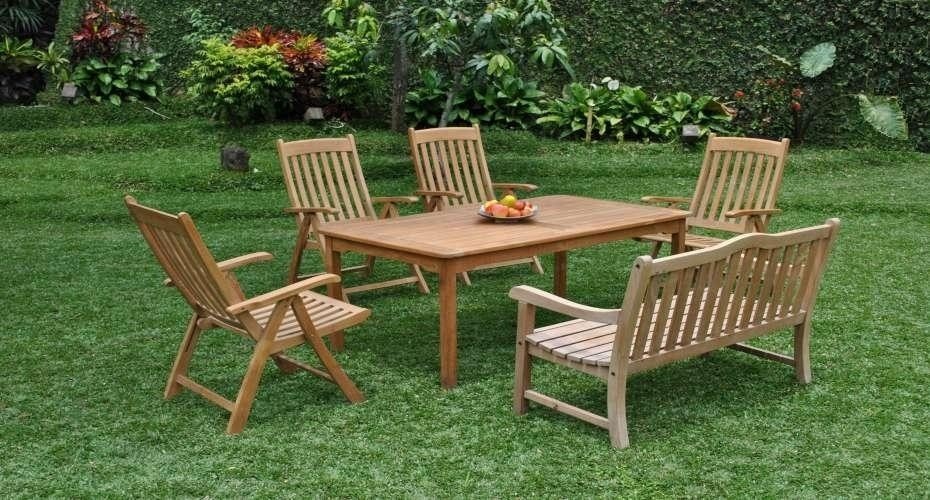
[123,193,374,434]
[273,131,430,304]
[413,121,545,286]
[638,130,790,304]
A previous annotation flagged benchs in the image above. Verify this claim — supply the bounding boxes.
[506,218,839,449]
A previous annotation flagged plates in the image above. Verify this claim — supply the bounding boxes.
[477,205,538,223]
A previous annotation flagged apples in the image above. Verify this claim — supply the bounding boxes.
[484,195,532,217]
